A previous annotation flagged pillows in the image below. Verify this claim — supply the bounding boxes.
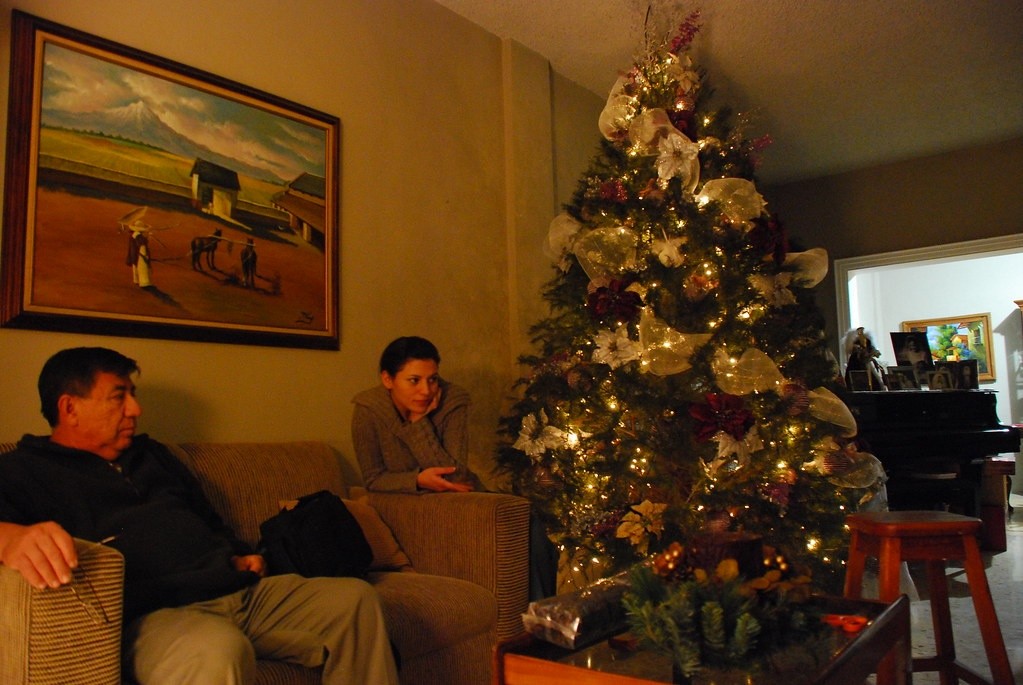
[277,486,410,570]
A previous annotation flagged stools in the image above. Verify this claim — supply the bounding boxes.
[843,511,1017,685]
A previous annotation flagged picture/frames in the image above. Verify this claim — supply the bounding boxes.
[901,311,995,382]
[2,8,343,353]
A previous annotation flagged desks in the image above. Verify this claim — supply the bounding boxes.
[486,592,916,685]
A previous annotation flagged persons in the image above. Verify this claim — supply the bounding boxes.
[0,347,400,685]
[351,336,483,495]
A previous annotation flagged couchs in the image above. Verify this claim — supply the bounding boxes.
[0,436,532,685]
